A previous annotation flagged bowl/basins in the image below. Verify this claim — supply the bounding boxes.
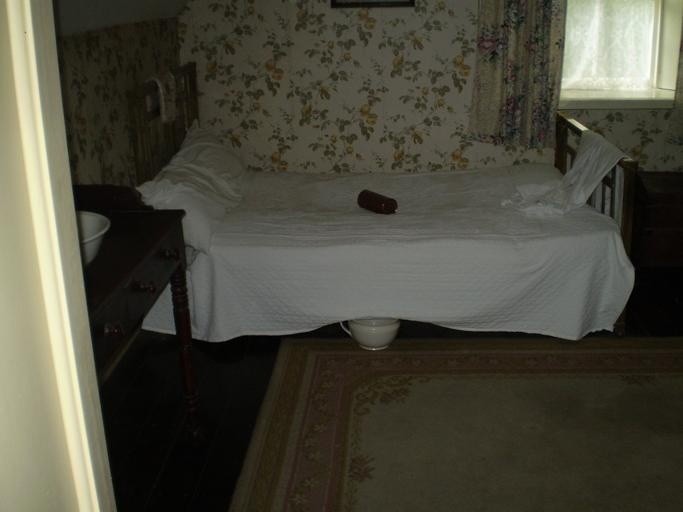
[75,210,111,270]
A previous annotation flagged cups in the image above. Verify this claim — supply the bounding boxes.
[339,311,401,351]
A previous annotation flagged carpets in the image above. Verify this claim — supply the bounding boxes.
[226,333,683,511]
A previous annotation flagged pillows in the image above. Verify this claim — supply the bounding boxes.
[133,119,254,255]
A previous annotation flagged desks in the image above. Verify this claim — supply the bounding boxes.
[80,209,198,407]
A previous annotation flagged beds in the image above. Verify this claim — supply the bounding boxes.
[123,62,638,337]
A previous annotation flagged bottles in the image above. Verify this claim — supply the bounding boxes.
[357,189,398,214]
